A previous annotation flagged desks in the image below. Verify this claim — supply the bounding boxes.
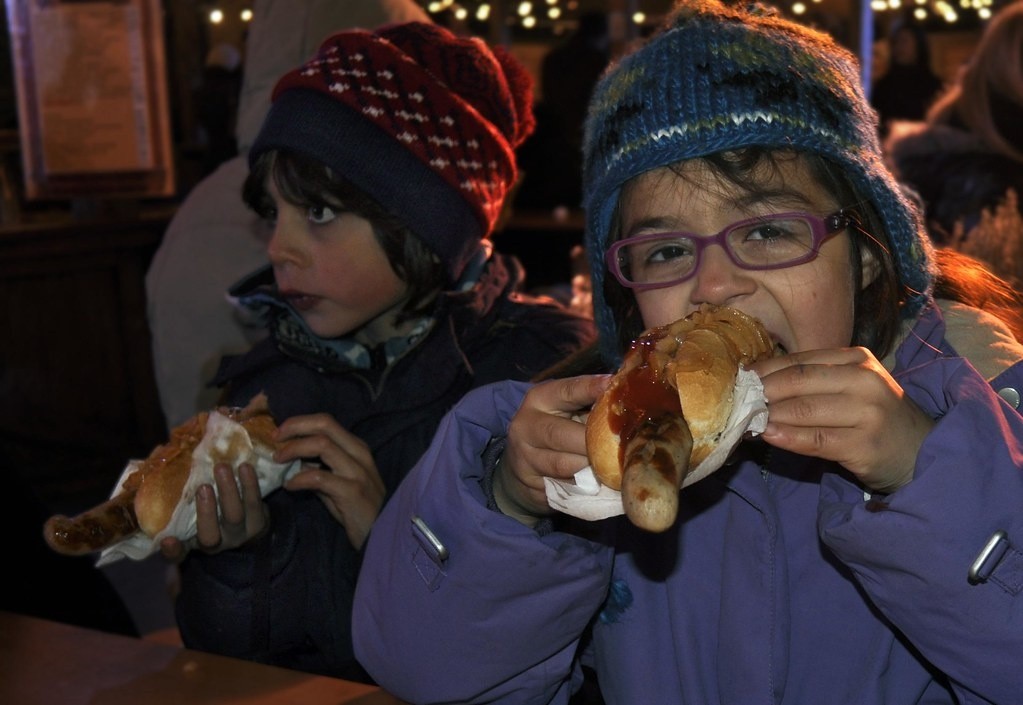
[0,607,396,705]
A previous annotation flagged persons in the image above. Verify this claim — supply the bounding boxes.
[153,21,603,689]
[491,0,1023,389]
[145,1,440,443]
[350,0,1023,705]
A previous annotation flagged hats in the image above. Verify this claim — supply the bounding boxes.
[584,0,931,340]
[267,20,532,241]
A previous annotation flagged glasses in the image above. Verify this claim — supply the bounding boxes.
[606,198,873,289]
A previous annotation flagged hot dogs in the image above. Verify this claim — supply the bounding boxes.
[46,410,280,557]
[588,307,774,533]
